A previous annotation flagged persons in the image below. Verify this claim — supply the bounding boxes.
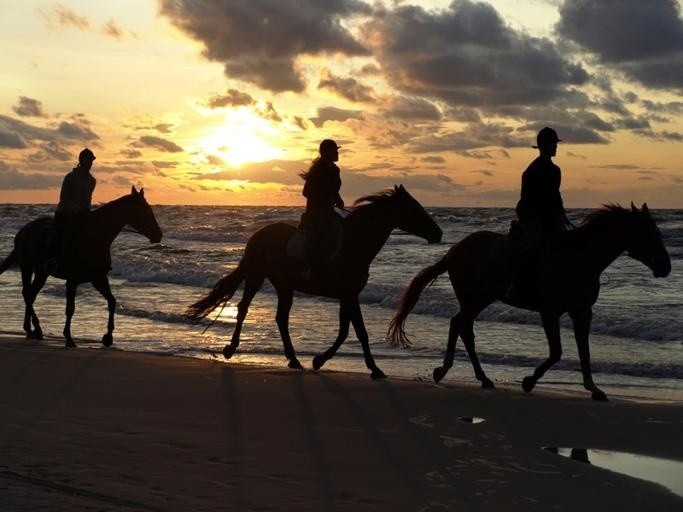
[503,125,567,305]
[297,138,348,297]
[44,148,97,276]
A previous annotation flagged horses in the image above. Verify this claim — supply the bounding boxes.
[182,184,443,379]
[0,186,163,349]
[386,201,671,401]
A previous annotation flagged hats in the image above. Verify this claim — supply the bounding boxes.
[319,139,341,153]
[537,126,561,145]
[79,149,96,162]
